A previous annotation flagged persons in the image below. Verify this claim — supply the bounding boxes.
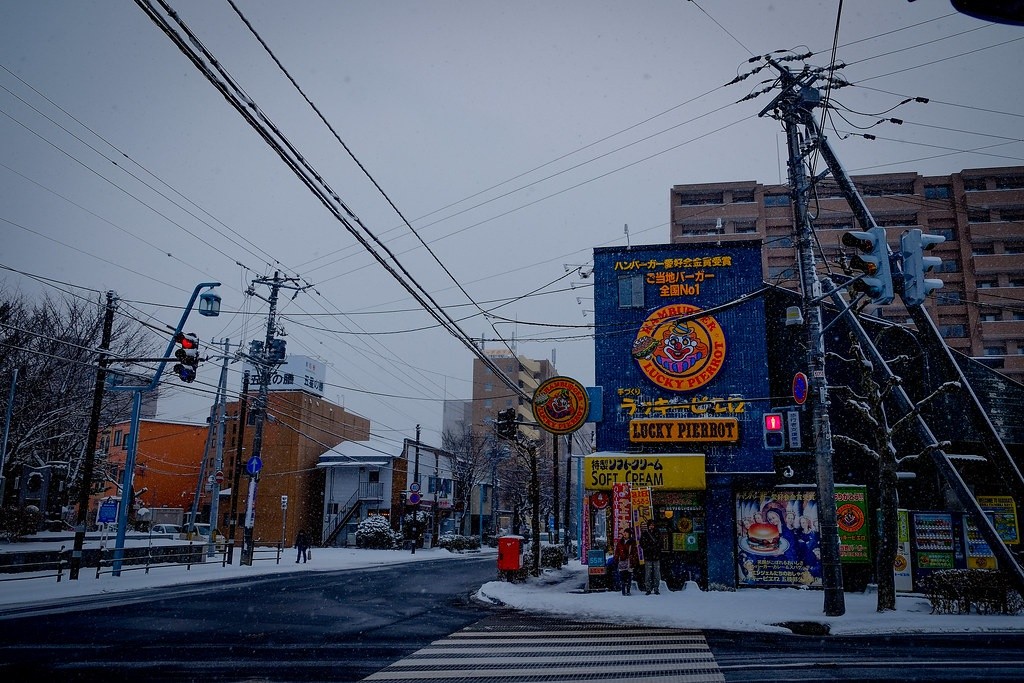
[563,532,572,555]
[737,501,820,585]
[639,518,664,596]
[605,546,614,592]
[614,528,639,596]
[293,529,312,564]
[523,530,530,553]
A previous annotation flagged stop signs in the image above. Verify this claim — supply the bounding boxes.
[215,471,225,485]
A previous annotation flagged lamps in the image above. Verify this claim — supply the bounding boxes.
[576,297,594,303]
[582,309,595,316]
[570,281,594,289]
[624,224,630,252]
[716,218,721,246]
[563,263,594,272]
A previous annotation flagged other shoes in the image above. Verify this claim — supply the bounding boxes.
[646,591,652,595]
[626,592,631,596]
[621,593,625,597]
[654,591,660,594]
[295,560,300,563]
[303,560,307,563]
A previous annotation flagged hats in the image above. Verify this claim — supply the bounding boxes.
[299,529,304,534]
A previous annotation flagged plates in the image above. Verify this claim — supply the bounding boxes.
[738,536,789,556]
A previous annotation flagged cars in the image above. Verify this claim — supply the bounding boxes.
[178,523,228,553]
[150,523,183,540]
[539,528,571,550]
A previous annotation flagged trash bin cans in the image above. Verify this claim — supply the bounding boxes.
[498,536,525,570]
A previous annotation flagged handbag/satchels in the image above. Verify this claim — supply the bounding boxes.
[308,548,312,560]
[617,558,632,572]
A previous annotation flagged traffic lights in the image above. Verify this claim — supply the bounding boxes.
[496,407,516,440]
[762,413,787,451]
[897,228,947,309]
[173,331,201,383]
[840,226,896,306]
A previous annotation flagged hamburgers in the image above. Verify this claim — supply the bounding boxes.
[746,522,782,551]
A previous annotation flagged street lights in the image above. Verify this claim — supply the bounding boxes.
[104,283,222,576]
[2,359,37,478]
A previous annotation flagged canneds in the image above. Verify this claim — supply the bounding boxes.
[916,518,943,526]
